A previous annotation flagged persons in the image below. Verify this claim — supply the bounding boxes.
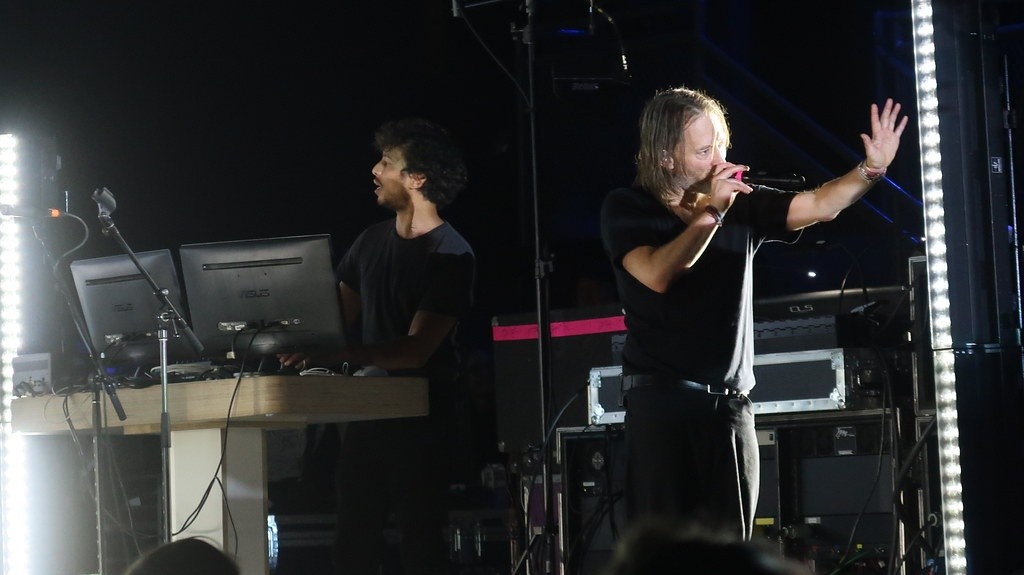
[277,118,492,575]
[600,87,908,542]
[124,538,241,575]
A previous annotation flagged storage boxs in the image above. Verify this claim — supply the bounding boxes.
[588,347,912,425]
[267,508,521,575]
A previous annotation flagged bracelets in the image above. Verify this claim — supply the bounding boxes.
[857,160,886,184]
[704,205,723,227]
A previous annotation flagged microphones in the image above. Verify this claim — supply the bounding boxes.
[723,168,806,188]
[0,204,66,217]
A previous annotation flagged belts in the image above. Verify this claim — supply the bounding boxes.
[624,375,740,398]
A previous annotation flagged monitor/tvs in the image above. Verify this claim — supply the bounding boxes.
[71,248,200,365]
[180,233,344,375]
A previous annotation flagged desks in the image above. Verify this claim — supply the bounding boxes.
[12,377,431,575]
